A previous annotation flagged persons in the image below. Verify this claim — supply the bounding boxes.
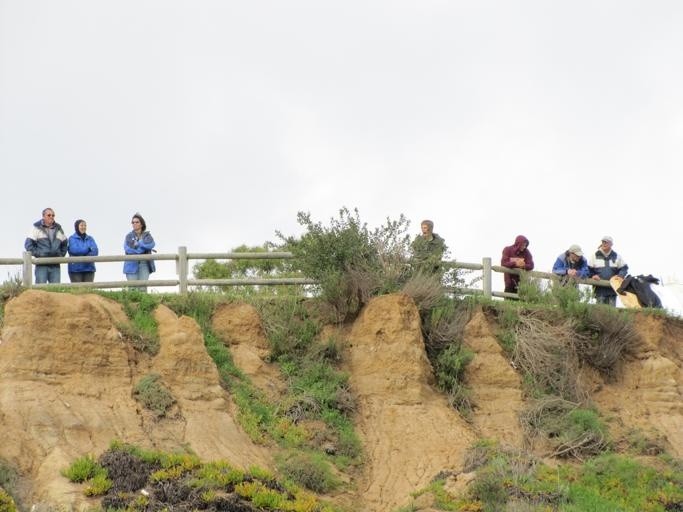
[67,219,98,282]
[552,245,589,291]
[123,213,157,293]
[24,209,69,283]
[501,235,534,300]
[413,220,445,284]
[589,235,628,307]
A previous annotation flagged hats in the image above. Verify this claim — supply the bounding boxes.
[569,245,584,257]
[601,236,612,242]
